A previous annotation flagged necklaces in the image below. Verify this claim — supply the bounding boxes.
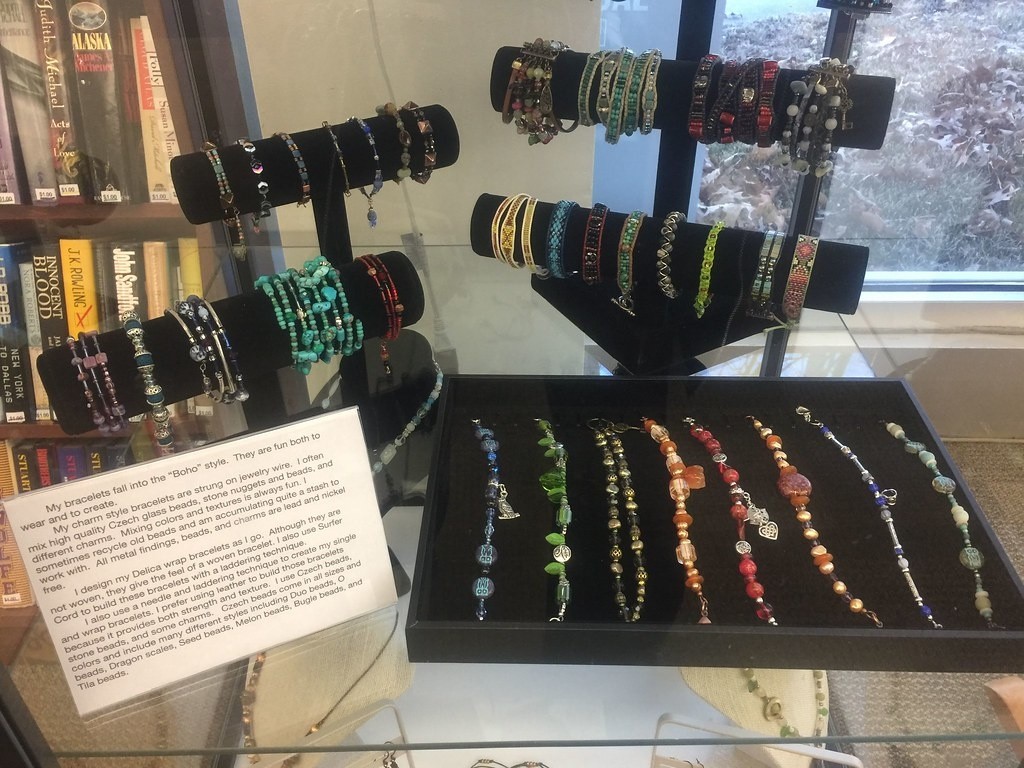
[237,610,402,768]
[321,359,445,478]
[472,405,1006,635]
[739,666,829,749]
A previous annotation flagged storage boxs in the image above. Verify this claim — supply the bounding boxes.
[404,374,1024,673]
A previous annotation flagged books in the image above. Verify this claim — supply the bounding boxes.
[0,0,193,207]
[0,236,215,426]
[0,436,204,611]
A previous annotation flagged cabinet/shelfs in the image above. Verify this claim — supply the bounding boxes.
[0,0,288,673]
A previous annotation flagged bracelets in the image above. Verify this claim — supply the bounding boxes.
[62,255,405,455]
[492,38,857,334]
[200,98,435,262]
